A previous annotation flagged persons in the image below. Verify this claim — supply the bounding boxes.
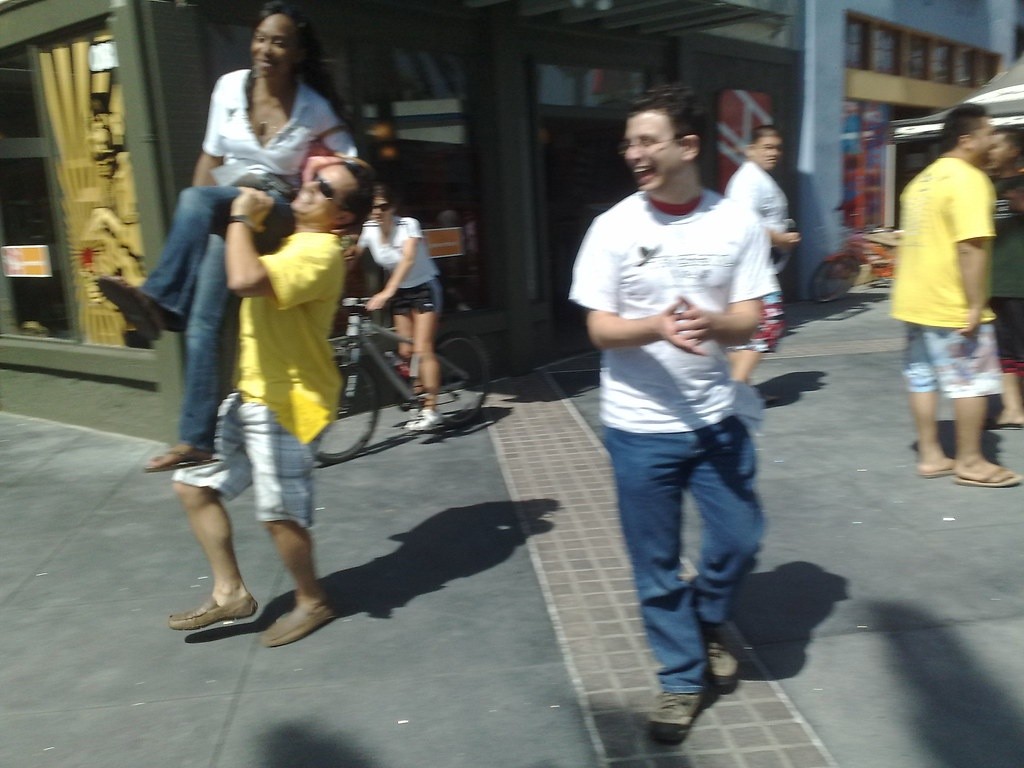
[567,89,781,743]
[888,102,1024,488]
[97,0,361,472]
[165,162,376,649]
[344,180,446,432]
[723,125,801,383]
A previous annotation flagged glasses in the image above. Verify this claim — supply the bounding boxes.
[310,173,334,201]
[615,135,682,153]
[369,203,392,213]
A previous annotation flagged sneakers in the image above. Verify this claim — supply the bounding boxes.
[643,693,707,746]
[406,409,446,430]
[699,625,741,685]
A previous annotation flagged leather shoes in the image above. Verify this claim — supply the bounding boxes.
[260,599,341,646]
[168,592,258,630]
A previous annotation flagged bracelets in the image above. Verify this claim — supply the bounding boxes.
[228,213,254,228]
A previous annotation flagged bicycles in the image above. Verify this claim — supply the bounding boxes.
[314,298,488,463]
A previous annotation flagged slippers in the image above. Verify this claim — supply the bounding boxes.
[142,448,216,472]
[96,274,159,340]
[951,460,1022,490]
[917,456,958,483]
